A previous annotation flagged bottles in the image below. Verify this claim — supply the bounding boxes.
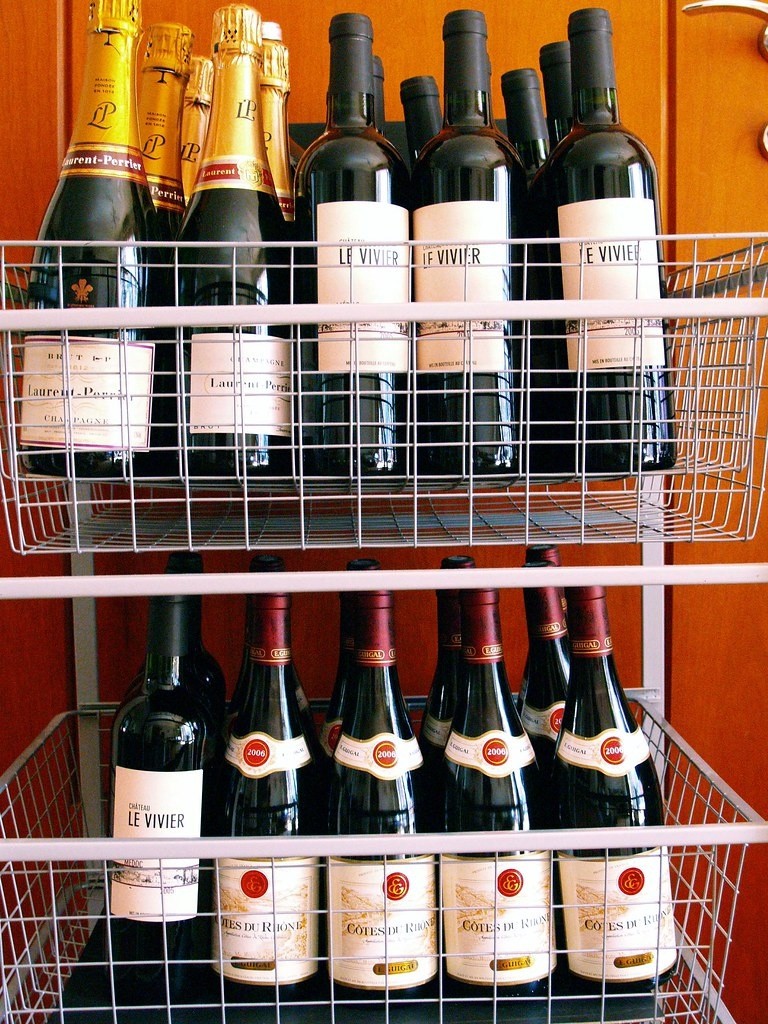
[100,546,679,1007]
[18,1,676,476]
